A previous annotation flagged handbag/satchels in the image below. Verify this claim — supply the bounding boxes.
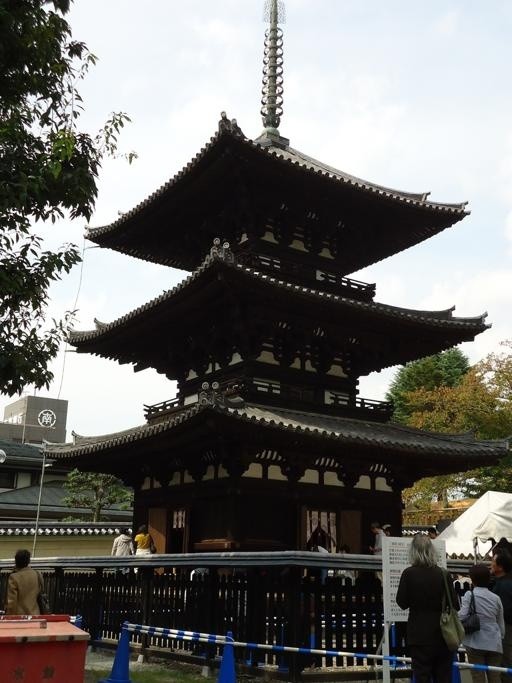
[147,537,156,552]
[461,614,480,635]
[37,589,50,615]
[440,609,465,652]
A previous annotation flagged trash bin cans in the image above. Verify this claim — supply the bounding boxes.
[2,614,89,683]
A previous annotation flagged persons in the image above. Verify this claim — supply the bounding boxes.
[368,522,387,596]
[396,535,460,683]
[111,528,134,575]
[134,525,154,575]
[5,548,45,616]
[457,564,506,683]
[335,544,359,597]
[490,548,512,683]
